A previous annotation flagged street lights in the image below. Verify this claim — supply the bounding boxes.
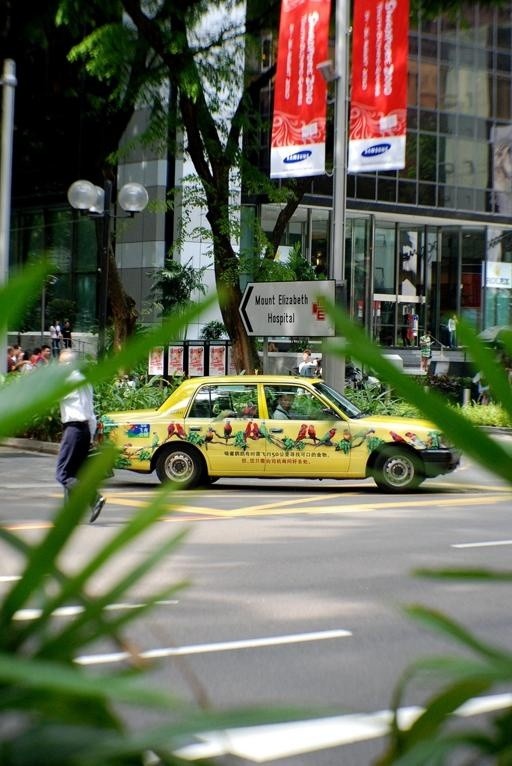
[67,176,149,361]
[40,274,58,347]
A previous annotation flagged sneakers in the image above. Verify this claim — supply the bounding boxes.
[90,497,106,523]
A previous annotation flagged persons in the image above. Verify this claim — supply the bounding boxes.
[50,320,63,357]
[448,314,459,348]
[273,395,293,420]
[61,322,72,348]
[402,308,413,346]
[299,349,322,379]
[345,355,354,379]
[56,349,105,523]
[419,331,434,372]
[7,344,51,372]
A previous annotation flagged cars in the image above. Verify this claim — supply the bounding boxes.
[96,375,461,493]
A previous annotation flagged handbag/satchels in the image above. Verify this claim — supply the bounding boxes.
[87,444,115,480]
